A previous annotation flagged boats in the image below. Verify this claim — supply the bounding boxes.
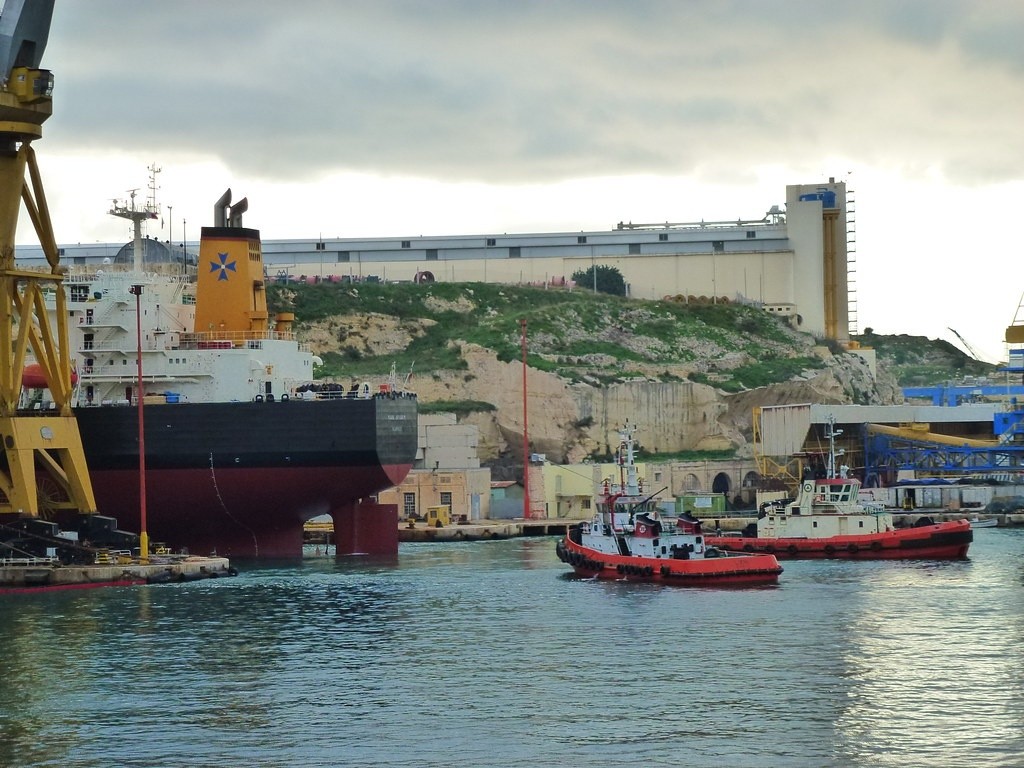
[703,414,973,559]
[556,417,784,583]
[1,550,240,581]
[25,160,418,557]
